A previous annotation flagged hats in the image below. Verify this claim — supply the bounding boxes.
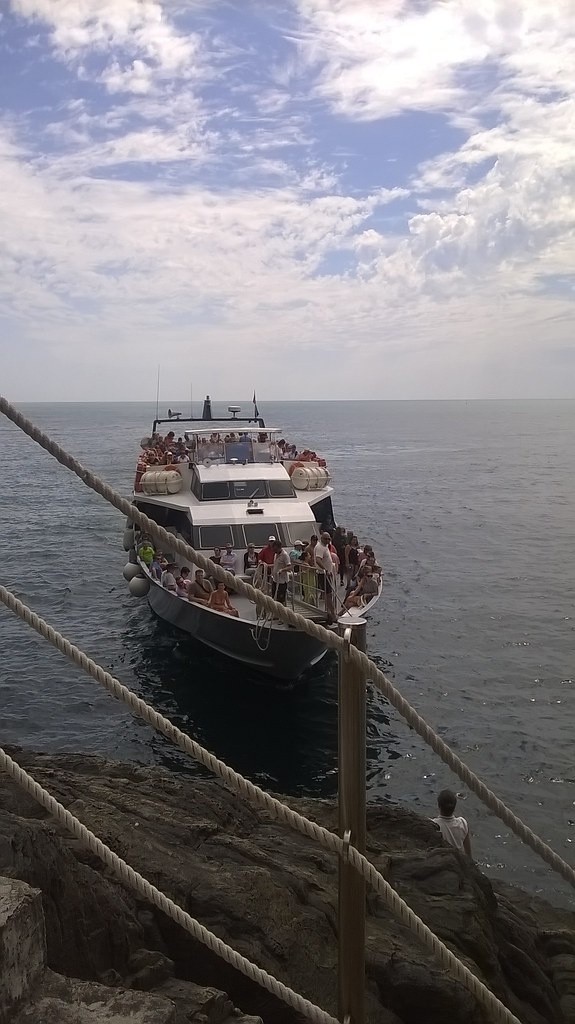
[294,539,307,546]
[269,536,276,541]
[226,543,234,548]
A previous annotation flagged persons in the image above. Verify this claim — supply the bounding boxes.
[289,532,340,623]
[208,583,239,618]
[319,514,382,616]
[175,576,192,598]
[268,541,292,625]
[243,543,259,576]
[258,536,276,564]
[220,543,237,576]
[189,570,213,606]
[161,564,177,592]
[428,790,472,859]
[137,535,155,568]
[276,439,312,462]
[176,566,191,592]
[203,546,223,585]
[150,553,163,582]
[146,431,190,465]
[210,432,268,443]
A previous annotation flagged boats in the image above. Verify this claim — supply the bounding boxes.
[133,363,382,673]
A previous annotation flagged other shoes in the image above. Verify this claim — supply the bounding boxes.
[319,595,322,599]
[340,581,344,586]
[338,612,344,616]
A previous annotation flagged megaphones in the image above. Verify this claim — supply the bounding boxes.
[168,409,182,418]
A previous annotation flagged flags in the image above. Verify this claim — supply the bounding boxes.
[253,392,259,417]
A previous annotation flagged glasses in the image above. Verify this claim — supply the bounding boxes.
[364,548,368,550]
[157,558,162,560]
[158,554,163,556]
[196,575,204,577]
[323,538,330,541]
[215,550,220,552]
[366,575,373,578]
[249,547,255,549]
[341,531,345,532]
[174,567,179,569]
[313,539,318,541]
[366,554,370,557]
